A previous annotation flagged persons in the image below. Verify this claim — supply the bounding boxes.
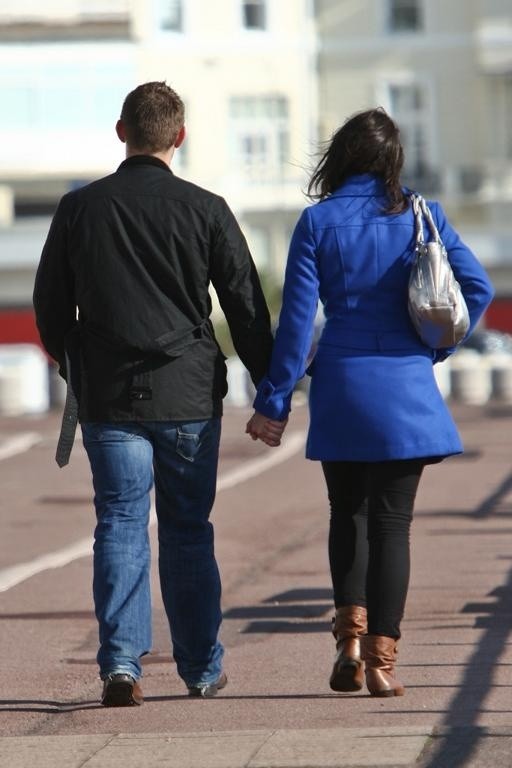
[33,82,287,706]
[246,106,495,697]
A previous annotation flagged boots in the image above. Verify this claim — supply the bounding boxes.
[330,606,403,697]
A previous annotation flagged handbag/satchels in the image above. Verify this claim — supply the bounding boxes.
[407,193,470,349]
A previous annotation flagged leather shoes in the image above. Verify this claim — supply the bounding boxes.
[188,672,227,699]
[99,673,143,706]
